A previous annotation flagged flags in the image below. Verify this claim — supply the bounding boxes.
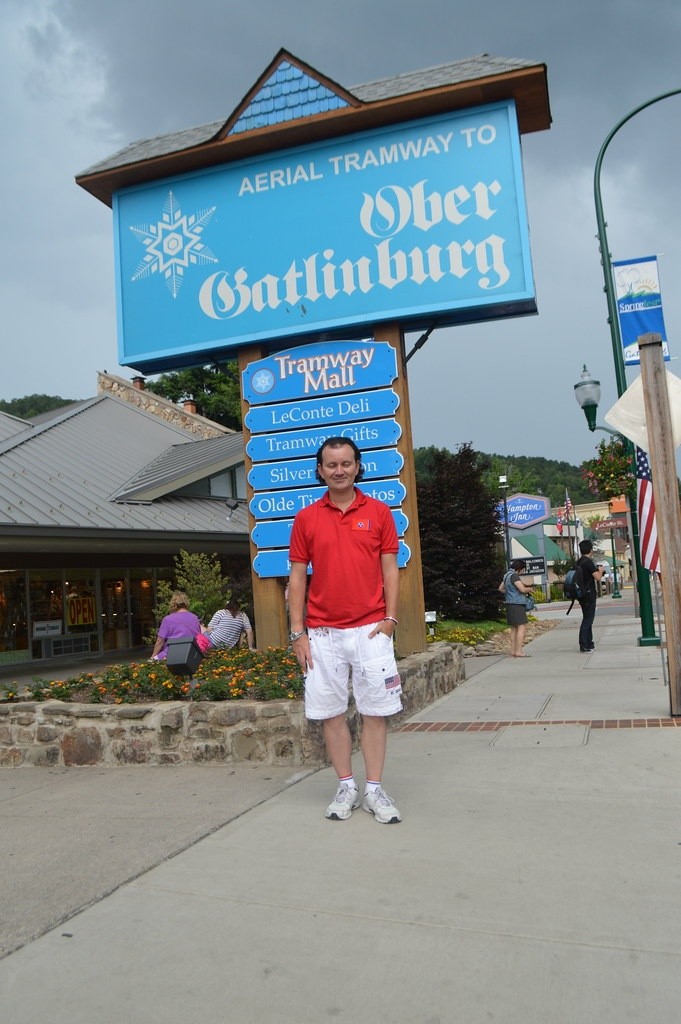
[635,445,663,584]
[563,488,572,522]
[555,505,563,534]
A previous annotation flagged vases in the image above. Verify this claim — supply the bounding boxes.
[608,494,627,515]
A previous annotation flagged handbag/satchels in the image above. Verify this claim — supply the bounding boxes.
[238,614,246,649]
[197,634,210,656]
[526,595,535,610]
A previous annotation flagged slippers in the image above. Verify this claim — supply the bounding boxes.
[513,651,531,657]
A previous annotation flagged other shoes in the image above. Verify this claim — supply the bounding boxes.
[580,643,595,653]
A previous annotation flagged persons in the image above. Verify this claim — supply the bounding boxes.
[498,560,535,657]
[147,590,202,661]
[199,598,257,655]
[287,437,402,824]
[610,568,621,592]
[575,540,604,654]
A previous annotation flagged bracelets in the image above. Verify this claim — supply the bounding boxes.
[249,646,253,650]
[384,615,399,625]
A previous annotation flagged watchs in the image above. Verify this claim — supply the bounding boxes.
[290,630,307,641]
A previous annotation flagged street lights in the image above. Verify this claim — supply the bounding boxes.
[572,363,664,647]
[497,475,510,571]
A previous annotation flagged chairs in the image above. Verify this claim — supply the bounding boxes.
[597,576,624,597]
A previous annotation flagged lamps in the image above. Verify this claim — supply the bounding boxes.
[166,637,206,682]
[424,611,438,636]
[224,497,239,521]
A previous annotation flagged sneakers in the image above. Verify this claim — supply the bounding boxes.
[359,781,402,823]
[325,783,361,819]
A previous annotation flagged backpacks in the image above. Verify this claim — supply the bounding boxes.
[564,558,588,599]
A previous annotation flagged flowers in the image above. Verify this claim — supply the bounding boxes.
[578,439,637,509]
